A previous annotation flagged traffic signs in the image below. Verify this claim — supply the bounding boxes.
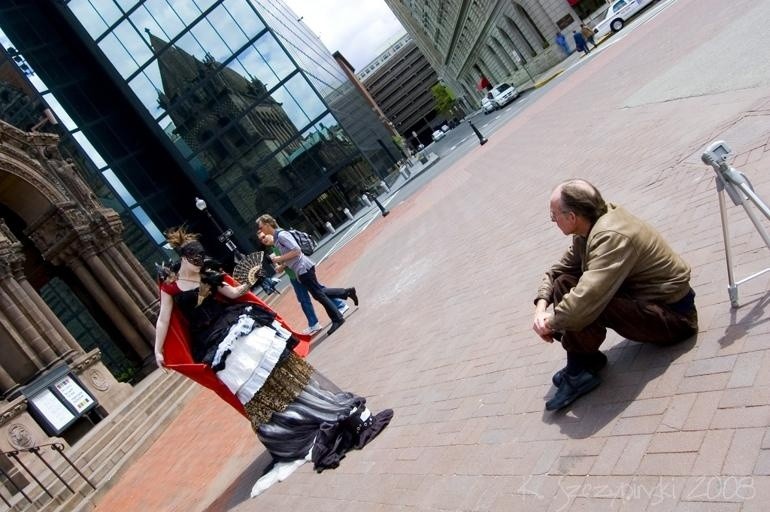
[219,229,234,242]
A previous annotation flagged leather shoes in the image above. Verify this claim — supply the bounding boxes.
[346,286,358,306]
[327,318,345,336]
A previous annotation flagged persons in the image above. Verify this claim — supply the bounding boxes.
[572,30,590,55]
[556,31,572,56]
[257,229,349,335]
[154,231,354,460]
[258,276,282,295]
[533,178,698,410]
[256,214,358,334]
[580,24,598,48]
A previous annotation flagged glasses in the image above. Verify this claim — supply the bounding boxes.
[258,234,266,241]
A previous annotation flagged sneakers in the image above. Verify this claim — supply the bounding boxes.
[552,348,609,387]
[302,321,322,337]
[543,368,601,411]
[337,305,349,313]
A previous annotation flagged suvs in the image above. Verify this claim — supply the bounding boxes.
[486,82,519,111]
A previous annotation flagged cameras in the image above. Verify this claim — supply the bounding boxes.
[701,140,730,169]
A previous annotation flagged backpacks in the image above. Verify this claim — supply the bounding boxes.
[277,228,318,257]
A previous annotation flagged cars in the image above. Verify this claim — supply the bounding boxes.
[417,117,461,151]
[479,96,495,115]
[591,1,653,40]
[359,172,385,196]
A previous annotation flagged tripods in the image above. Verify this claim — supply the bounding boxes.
[715,169,770,306]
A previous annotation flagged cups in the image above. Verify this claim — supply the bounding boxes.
[270,262,280,271]
[267,251,276,260]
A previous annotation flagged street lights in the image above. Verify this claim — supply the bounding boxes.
[195,197,275,295]
